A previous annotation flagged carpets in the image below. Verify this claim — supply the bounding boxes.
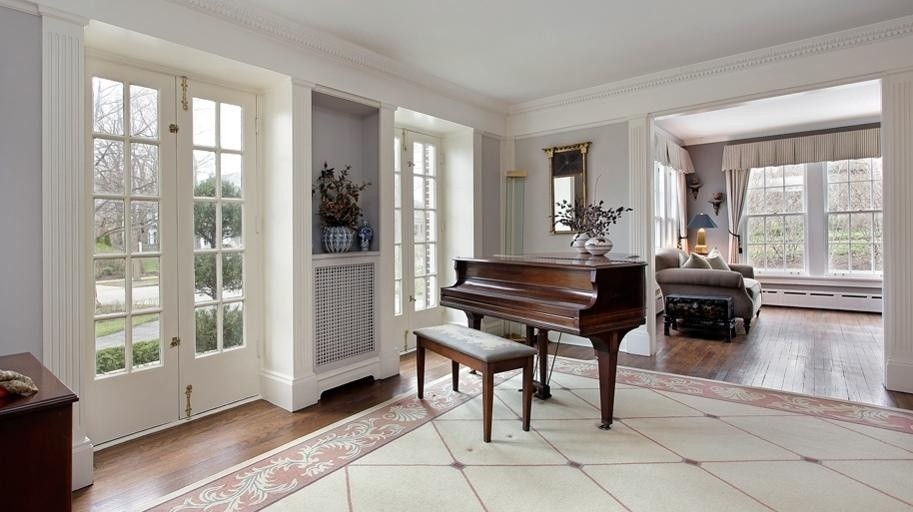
[141,353,911,512]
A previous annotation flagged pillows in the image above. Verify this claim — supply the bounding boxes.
[679,252,712,269]
[703,245,731,270]
[678,250,688,268]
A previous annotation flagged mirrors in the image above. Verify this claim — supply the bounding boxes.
[540,139,592,235]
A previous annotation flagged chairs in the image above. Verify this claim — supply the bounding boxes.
[654,247,762,335]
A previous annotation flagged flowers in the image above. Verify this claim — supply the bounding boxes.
[546,196,633,248]
[311,160,372,228]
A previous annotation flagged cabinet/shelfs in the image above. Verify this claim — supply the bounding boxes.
[1,350,79,512]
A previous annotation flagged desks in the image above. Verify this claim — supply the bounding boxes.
[662,292,736,343]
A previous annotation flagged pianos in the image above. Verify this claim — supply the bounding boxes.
[440,250,648,431]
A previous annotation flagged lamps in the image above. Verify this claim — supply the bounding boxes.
[687,212,718,254]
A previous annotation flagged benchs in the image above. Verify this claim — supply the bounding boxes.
[411,322,537,444]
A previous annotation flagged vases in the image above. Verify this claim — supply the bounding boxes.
[320,221,355,253]
[571,231,595,254]
[583,235,614,257]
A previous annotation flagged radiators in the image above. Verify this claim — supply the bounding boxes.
[760,287,882,316]
